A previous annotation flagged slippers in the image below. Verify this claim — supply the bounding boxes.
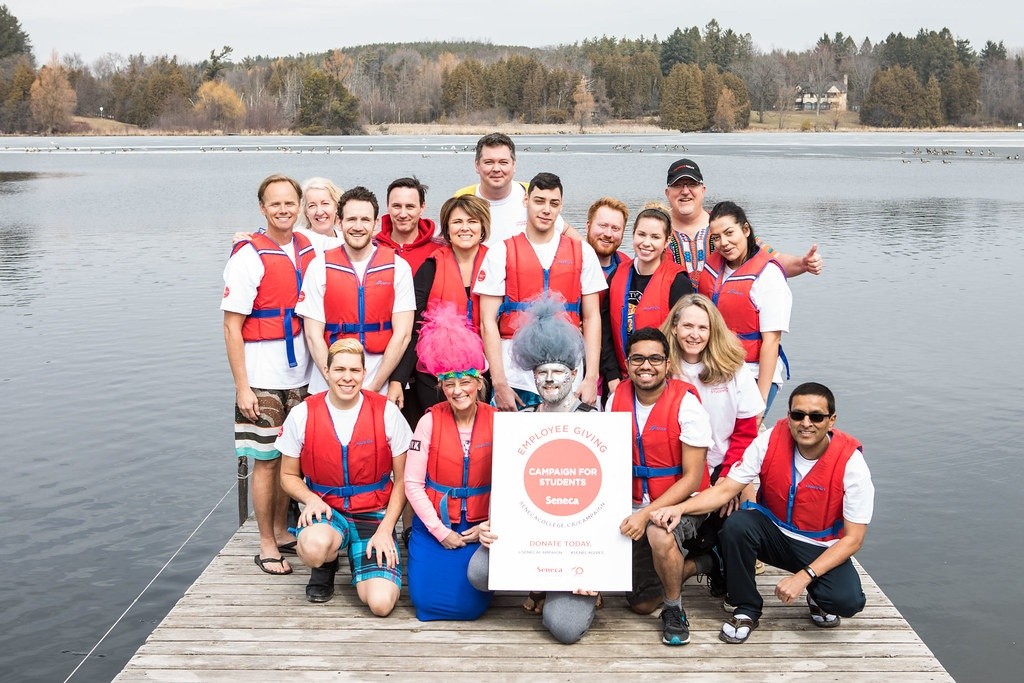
[720,616,759,643]
[278,541,297,553]
[522,591,545,615]
[255,555,292,574]
[807,593,841,628]
[595,593,604,610]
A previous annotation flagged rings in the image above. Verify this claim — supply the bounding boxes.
[389,548,396,554]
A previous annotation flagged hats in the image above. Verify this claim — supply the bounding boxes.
[667,159,703,186]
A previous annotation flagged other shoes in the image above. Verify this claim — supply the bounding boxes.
[402,525,411,548]
[724,591,738,611]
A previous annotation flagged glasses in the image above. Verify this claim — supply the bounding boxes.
[628,354,667,366]
[790,411,831,422]
[673,182,699,189]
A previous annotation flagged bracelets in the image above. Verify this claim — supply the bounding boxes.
[800,565,819,582]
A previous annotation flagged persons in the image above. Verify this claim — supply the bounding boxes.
[656,294,768,612]
[403,299,499,622]
[467,289,600,644]
[296,131,823,552]
[647,382,875,645]
[273,338,414,616]
[218,175,316,575]
[604,326,716,644]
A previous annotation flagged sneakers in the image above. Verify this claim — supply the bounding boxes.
[707,544,727,597]
[658,605,690,644]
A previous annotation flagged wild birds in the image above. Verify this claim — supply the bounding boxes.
[901,146,1019,164]
[3,144,691,157]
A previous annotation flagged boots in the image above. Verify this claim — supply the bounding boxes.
[306,553,340,601]
